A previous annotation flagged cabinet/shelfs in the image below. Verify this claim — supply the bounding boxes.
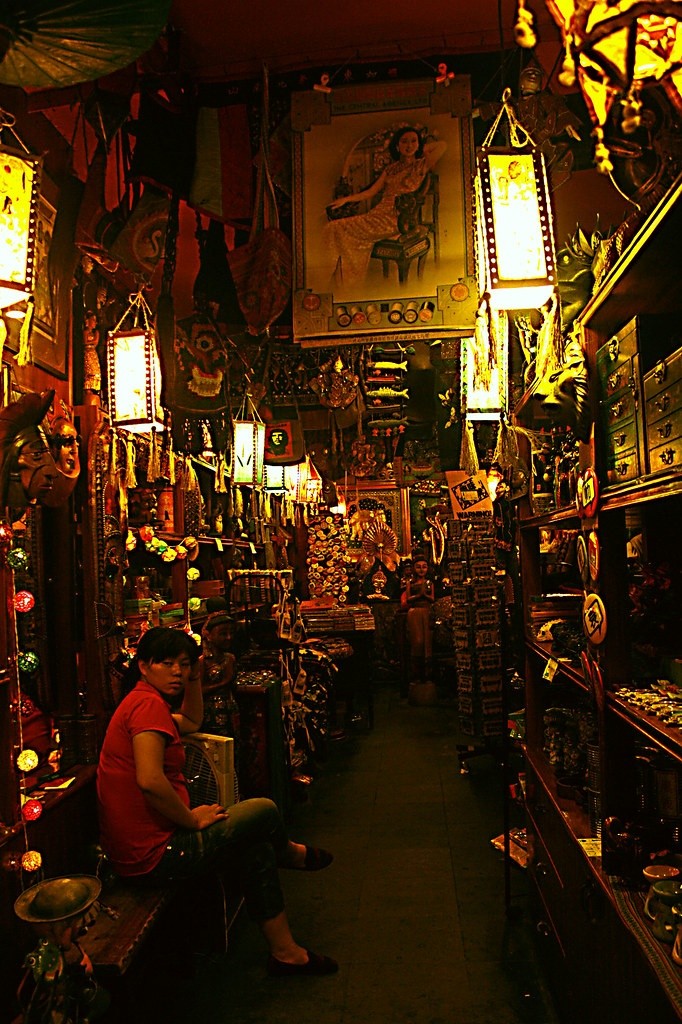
[513,172,682,1024]
[71,395,299,760]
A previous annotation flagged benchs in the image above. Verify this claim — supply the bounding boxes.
[76,864,230,979]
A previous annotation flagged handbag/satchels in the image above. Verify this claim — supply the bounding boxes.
[256,420,306,465]
[227,228,295,336]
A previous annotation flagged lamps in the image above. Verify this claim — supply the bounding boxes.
[0,109,44,365]
[107,283,164,489]
[460,311,541,474]
[234,390,266,519]
[474,103,563,385]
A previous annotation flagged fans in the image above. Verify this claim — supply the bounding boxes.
[182,732,238,811]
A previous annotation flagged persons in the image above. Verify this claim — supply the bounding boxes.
[96,626,337,977]
[406,555,436,687]
[199,596,251,802]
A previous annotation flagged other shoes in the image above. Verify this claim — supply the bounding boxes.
[298,845,335,872]
[266,952,338,976]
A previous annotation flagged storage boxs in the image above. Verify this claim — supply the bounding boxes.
[596,314,682,484]
[445,519,503,736]
